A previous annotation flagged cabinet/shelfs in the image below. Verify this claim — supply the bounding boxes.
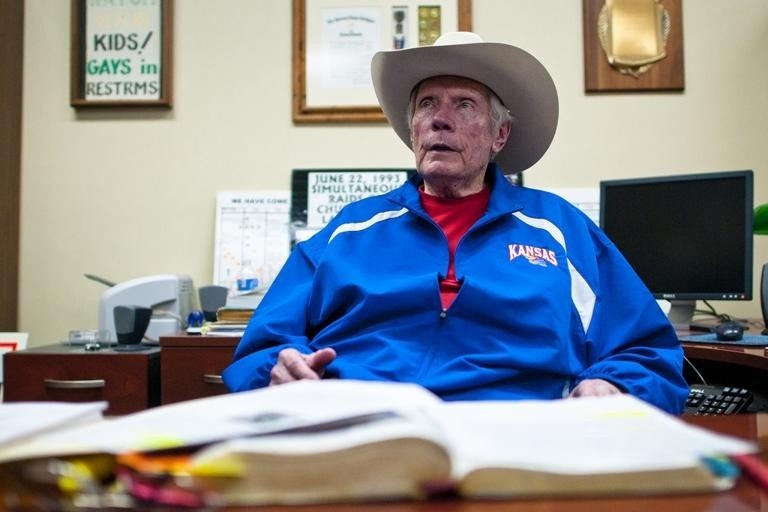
[1,345,150,414]
[155,311,243,409]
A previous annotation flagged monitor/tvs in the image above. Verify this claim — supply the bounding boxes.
[600,169,754,336]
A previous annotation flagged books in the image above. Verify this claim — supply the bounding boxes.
[2,396,109,469]
[215,294,266,322]
[199,322,249,338]
[189,391,756,505]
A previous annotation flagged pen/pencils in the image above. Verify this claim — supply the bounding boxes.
[731,454,768,494]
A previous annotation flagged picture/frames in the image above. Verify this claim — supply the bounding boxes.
[290,1,475,124]
[65,1,175,111]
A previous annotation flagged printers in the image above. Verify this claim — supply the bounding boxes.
[65,271,194,345]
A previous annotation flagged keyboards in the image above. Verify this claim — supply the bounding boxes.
[682,383,753,416]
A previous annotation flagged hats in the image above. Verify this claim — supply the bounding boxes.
[371,31,558,176]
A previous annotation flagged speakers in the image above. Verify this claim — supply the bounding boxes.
[198,285,229,322]
[112,303,154,351]
[760,264,768,335]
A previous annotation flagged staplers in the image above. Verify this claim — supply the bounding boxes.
[185,310,203,334]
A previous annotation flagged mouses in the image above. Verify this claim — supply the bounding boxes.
[715,322,744,341]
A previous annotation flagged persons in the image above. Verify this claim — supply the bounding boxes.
[223,31,688,418]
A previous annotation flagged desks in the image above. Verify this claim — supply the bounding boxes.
[672,319,767,383]
[1,396,767,512]
